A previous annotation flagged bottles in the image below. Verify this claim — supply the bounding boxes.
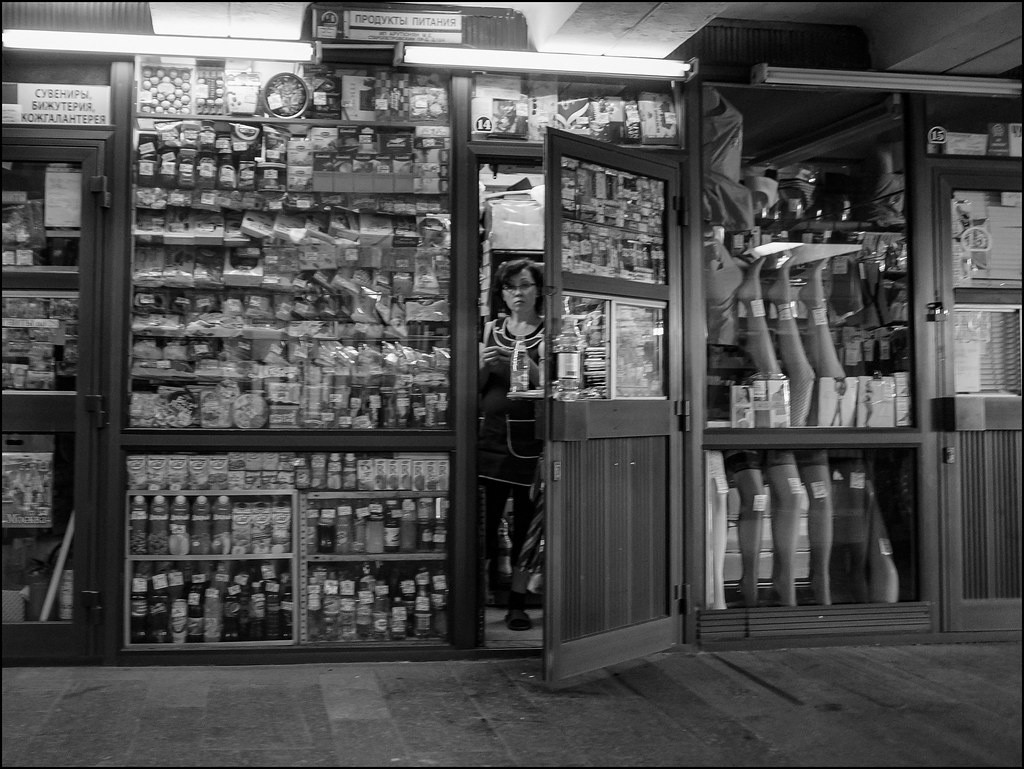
[307,497,448,553]
[327,453,342,490]
[307,561,446,640]
[343,452,356,490]
[553,315,582,401]
[130,495,232,555]
[130,561,293,640]
[510,334,529,392]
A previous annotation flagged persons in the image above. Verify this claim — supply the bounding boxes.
[477,259,565,631]
[702,85,908,609]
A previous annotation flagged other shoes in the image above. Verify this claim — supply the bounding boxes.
[506,613,532,630]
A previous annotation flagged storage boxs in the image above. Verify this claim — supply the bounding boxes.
[133,213,418,284]
[2,435,55,530]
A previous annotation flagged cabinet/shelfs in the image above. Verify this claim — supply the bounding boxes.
[123,488,450,651]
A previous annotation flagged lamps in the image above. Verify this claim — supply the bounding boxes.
[394,41,700,83]
[751,63,1024,97]
[0,30,323,65]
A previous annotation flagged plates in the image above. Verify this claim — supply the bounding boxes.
[263,72,309,119]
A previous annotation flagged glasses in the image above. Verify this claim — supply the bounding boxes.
[501,281,536,292]
